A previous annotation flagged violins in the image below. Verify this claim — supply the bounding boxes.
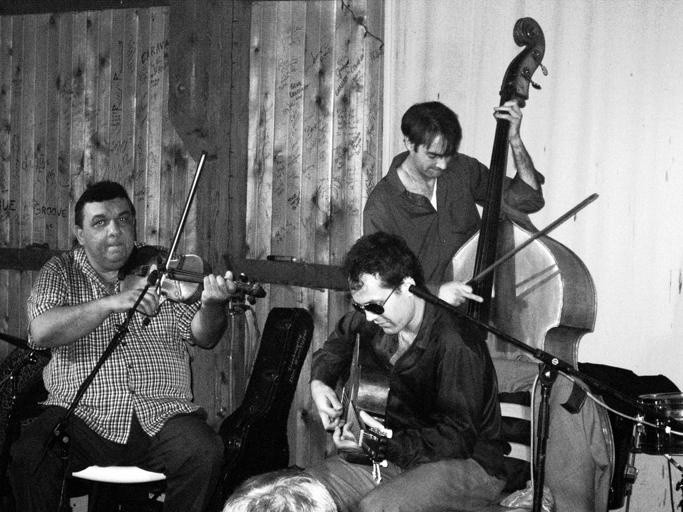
[118,245,266,316]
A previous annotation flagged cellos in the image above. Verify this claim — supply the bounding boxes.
[577,363,683,455]
[441,17,597,393]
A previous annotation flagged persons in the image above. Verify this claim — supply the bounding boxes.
[223,465,338,512]
[308,230,517,509]
[361,99,545,309]
[4,179,238,511]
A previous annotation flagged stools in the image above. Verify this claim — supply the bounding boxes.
[72,465,167,512]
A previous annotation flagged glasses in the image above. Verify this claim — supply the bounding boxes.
[352,280,403,314]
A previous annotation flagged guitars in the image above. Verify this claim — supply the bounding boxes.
[324,365,392,468]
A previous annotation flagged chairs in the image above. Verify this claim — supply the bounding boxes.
[479,357,607,511]
